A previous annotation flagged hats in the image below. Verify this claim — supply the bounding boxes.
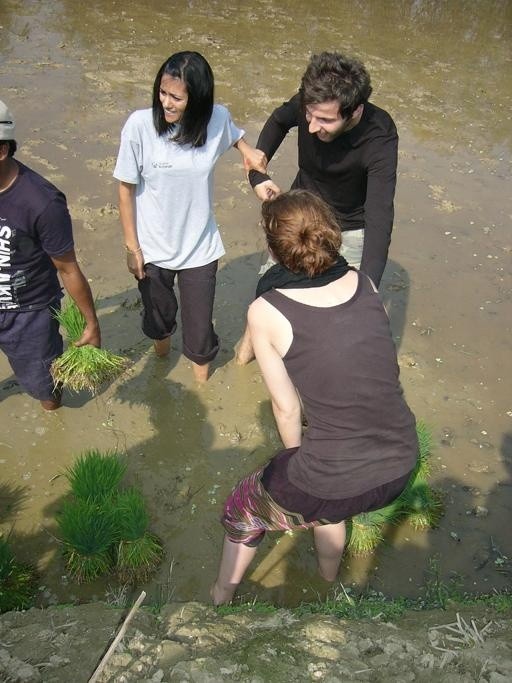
[0,101,15,141]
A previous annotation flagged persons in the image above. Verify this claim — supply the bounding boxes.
[0,95,102,410]
[111,51,270,384]
[210,187,422,607]
[234,51,401,366]
